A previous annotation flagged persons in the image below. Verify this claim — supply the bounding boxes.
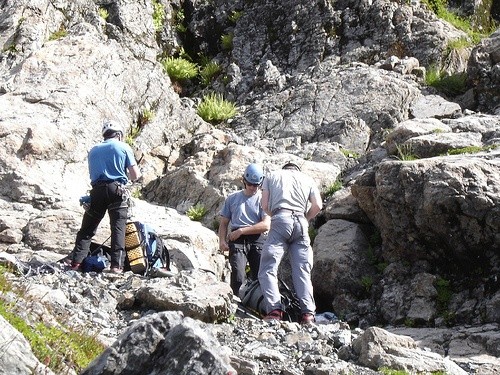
[67,121,139,275]
[257,160,322,323]
[218,163,270,296]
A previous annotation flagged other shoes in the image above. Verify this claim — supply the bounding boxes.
[71,263,80,270]
[264,310,284,320]
[302,313,315,323]
[110,268,123,273]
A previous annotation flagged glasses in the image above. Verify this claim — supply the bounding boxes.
[247,182,259,187]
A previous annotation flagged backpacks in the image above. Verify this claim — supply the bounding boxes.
[139,223,173,272]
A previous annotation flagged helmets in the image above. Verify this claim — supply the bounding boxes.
[243,164,263,184]
[282,161,301,171]
[102,121,124,137]
